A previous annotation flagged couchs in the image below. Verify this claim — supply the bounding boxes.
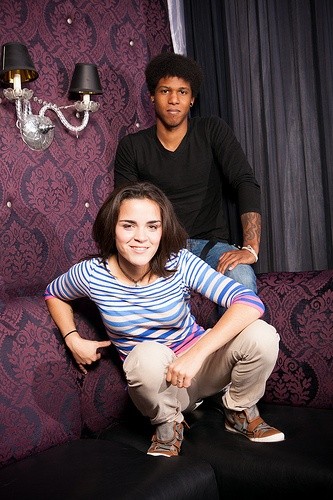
[0,270,333,500]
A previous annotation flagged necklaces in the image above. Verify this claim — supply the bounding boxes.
[116,253,152,286]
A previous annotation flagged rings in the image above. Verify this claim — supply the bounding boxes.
[178,380,183,383]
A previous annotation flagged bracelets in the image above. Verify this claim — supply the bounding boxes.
[62,330,78,339]
[241,245,258,264]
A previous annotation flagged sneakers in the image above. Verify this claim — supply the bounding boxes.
[224,404,285,442]
[147,420,190,458]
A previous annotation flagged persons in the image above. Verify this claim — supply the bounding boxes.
[113,53,262,314]
[43,183,286,457]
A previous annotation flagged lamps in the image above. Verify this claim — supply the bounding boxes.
[0,42,104,152]
